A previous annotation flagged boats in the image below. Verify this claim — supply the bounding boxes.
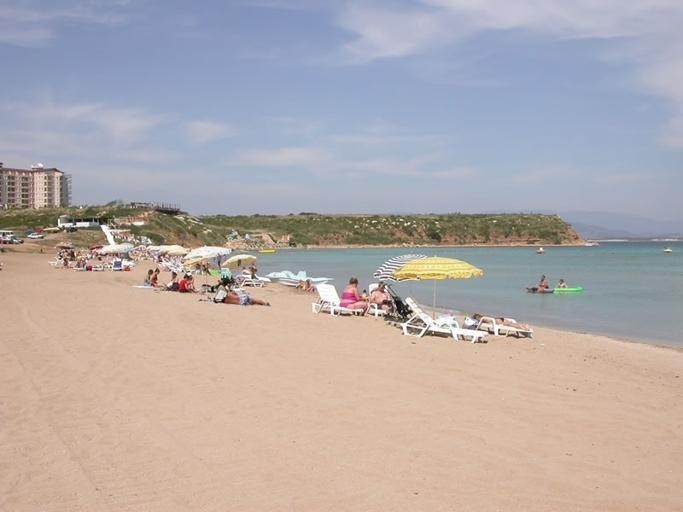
[664,248,672,252]
[279,278,333,287]
[526,287,582,293]
[258,250,276,253]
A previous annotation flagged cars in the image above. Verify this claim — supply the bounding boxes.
[0,231,24,244]
[30,233,43,239]
[43,226,60,232]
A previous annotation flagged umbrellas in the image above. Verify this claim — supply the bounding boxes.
[53,240,188,264]
[391,255,486,321]
[372,253,432,298]
[182,244,231,297]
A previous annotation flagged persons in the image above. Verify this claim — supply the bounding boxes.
[556,279,568,289]
[369,280,396,318]
[338,276,369,317]
[53,249,316,308]
[539,274,548,288]
[472,312,531,331]
[535,280,544,293]
[361,289,368,302]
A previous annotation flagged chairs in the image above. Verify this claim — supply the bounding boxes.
[221,267,271,289]
[307,281,531,343]
